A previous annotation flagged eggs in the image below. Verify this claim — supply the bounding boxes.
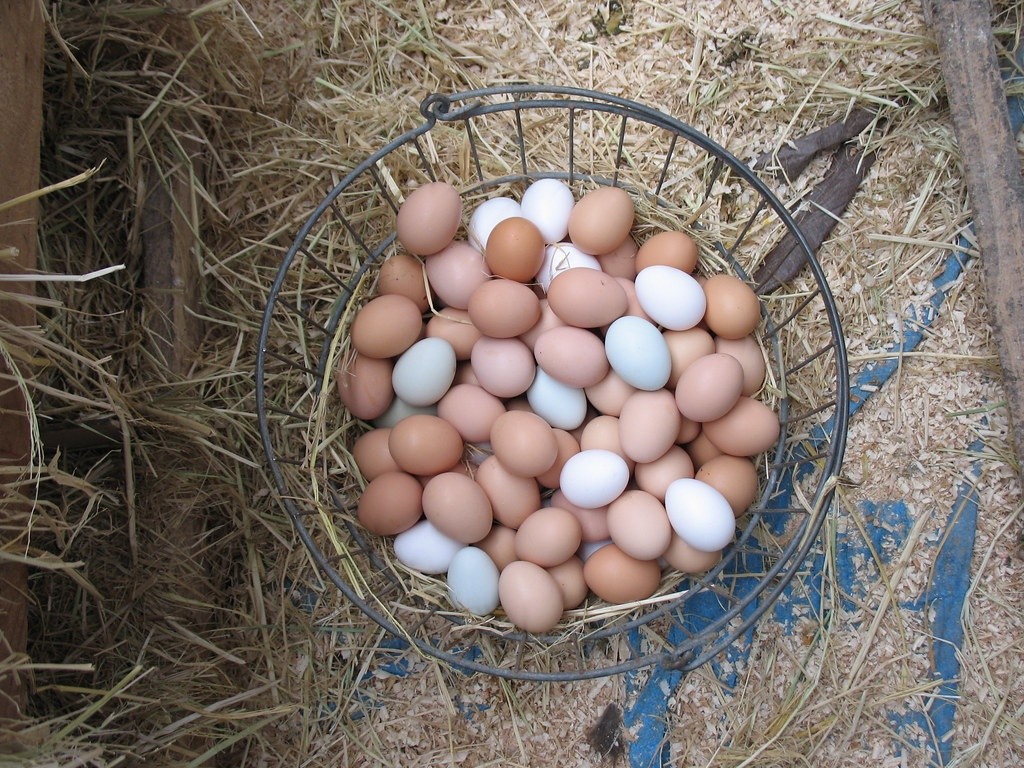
[335,178,780,632]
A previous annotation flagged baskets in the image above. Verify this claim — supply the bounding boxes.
[260,81,852,682]
